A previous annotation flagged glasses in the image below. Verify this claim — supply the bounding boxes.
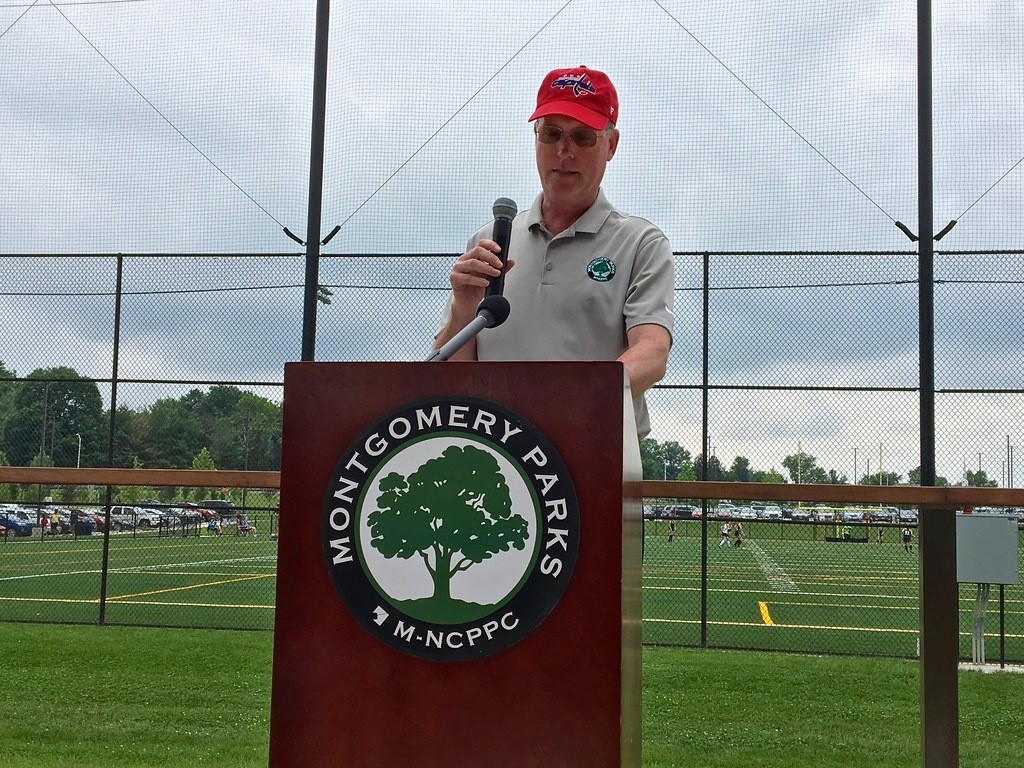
[535,124,611,147]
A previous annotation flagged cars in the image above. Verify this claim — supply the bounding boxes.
[643,502,1024,529]
[0,497,238,538]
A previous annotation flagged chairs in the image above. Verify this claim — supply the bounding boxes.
[235,524,251,538]
[208,525,217,536]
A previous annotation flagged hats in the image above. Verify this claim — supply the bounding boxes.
[528,65,619,130]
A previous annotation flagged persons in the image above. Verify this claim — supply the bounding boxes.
[40,509,60,538]
[668,513,914,553]
[434,65,676,440]
[209,515,258,537]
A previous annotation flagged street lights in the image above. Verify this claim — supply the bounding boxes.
[75,432,82,469]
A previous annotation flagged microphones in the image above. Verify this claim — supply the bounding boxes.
[484,197,518,298]
[430,296,511,362]
